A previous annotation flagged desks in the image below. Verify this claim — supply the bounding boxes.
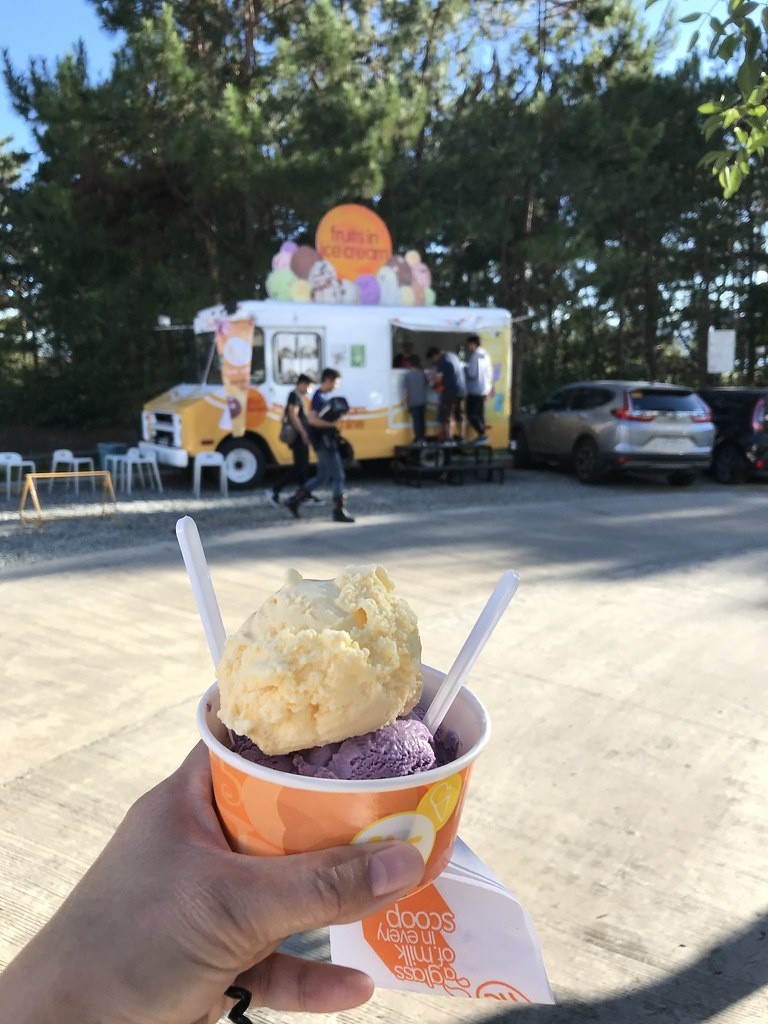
[394,441,494,486]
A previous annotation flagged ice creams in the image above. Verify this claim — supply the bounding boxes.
[212,561,466,784]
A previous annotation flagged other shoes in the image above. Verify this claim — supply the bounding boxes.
[285,496,300,517]
[333,507,355,522]
[411,438,427,447]
[265,487,281,509]
[438,439,457,448]
[301,496,327,506]
[471,434,490,447]
[458,439,466,447]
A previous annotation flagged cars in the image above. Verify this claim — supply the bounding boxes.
[697,386,768,485]
[509,379,717,489]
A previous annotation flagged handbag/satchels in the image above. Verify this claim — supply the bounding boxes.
[279,422,297,444]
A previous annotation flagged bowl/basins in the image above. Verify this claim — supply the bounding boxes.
[197,663,491,901]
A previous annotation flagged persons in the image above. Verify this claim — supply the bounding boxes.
[393,339,420,369]
[426,346,468,447]
[464,335,492,448]
[0,738,424,1024]
[265,374,326,508]
[282,367,355,522]
[404,356,428,444]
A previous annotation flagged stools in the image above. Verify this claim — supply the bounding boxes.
[0,447,230,515]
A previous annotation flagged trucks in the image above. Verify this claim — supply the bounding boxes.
[138,299,514,491]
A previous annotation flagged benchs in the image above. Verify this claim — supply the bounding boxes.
[391,458,504,488]
